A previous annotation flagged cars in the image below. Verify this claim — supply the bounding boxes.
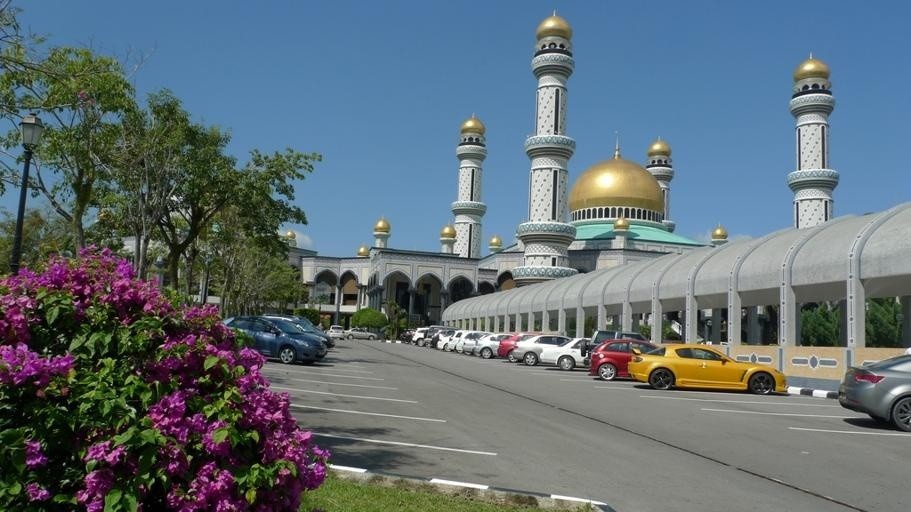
[344,327,378,341]
[837,354,911,434]
[220,314,336,364]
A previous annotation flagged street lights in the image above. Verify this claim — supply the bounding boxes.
[202,251,214,306]
[10,111,45,276]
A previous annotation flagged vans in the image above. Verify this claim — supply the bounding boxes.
[328,324,344,340]
